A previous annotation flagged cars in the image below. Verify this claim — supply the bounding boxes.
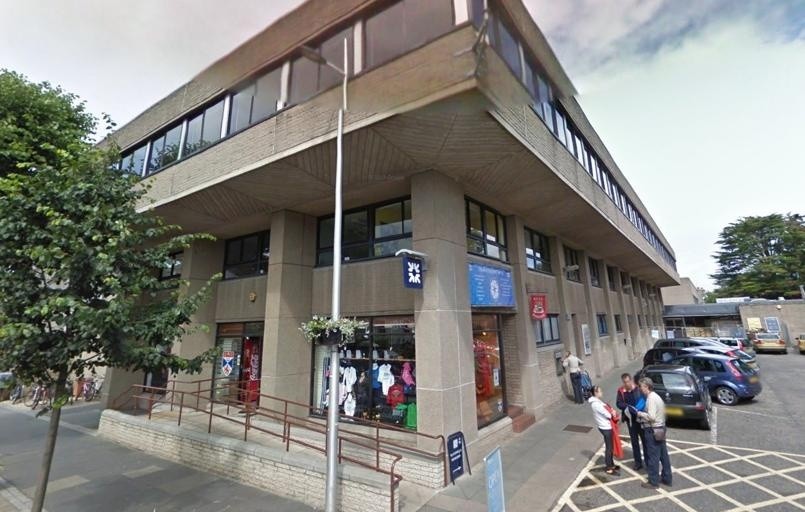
[752,332,786,354]
[794,332,805,353]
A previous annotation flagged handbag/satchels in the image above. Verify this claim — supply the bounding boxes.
[654,427,665,442]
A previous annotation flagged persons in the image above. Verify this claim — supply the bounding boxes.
[562,351,584,404]
[588,373,672,489]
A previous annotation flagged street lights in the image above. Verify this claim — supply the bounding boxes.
[298,37,348,512]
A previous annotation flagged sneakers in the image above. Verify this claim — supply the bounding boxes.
[641,477,672,489]
[634,462,642,470]
[606,465,620,475]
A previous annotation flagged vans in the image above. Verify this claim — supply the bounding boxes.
[634,337,762,430]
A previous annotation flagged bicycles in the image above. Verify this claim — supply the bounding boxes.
[10,374,105,410]
